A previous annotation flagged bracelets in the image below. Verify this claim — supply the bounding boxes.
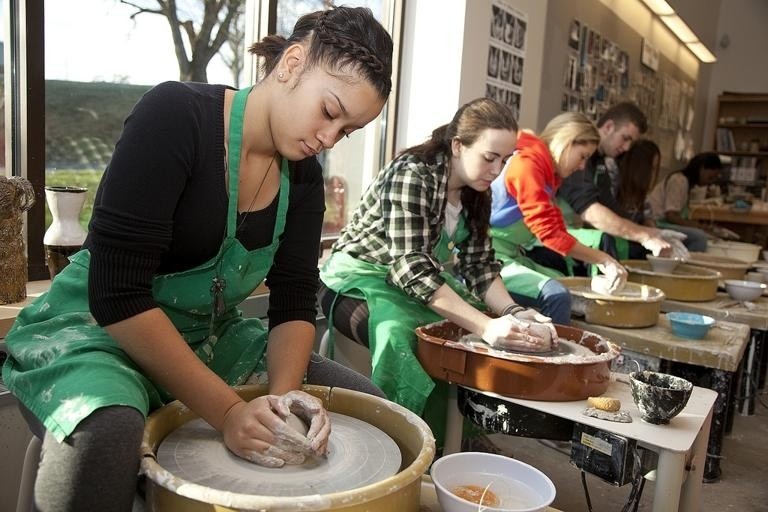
[500,305,525,316]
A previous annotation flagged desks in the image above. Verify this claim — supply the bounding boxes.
[440,364,718,512]
[566,309,754,484]
[658,272,767,423]
[414,471,566,512]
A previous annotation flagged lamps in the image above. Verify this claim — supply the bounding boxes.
[638,0,719,68]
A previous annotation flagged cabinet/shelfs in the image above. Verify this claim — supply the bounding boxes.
[710,88,767,203]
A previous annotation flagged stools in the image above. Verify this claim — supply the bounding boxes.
[319,328,377,381]
[14,403,47,512]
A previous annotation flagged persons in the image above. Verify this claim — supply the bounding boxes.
[1,6,394,512]
[488,111,628,325]
[735,139,763,199]
[646,152,740,253]
[315,97,558,457]
[596,139,660,259]
[527,103,691,278]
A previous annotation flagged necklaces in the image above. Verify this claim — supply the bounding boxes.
[445,203,463,250]
[209,149,278,318]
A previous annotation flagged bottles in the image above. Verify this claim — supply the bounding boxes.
[750,139,760,151]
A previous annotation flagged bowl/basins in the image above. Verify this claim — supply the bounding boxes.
[138,384,437,512]
[616,259,719,303]
[666,248,750,283]
[723,278,768,303]
[407,310,619,402]
[705,239,760,263]
[646,252,681,274]
[426,451,557,511]
[666,311,715,339]
[627,367,694,427]
[554,276,666,331]
